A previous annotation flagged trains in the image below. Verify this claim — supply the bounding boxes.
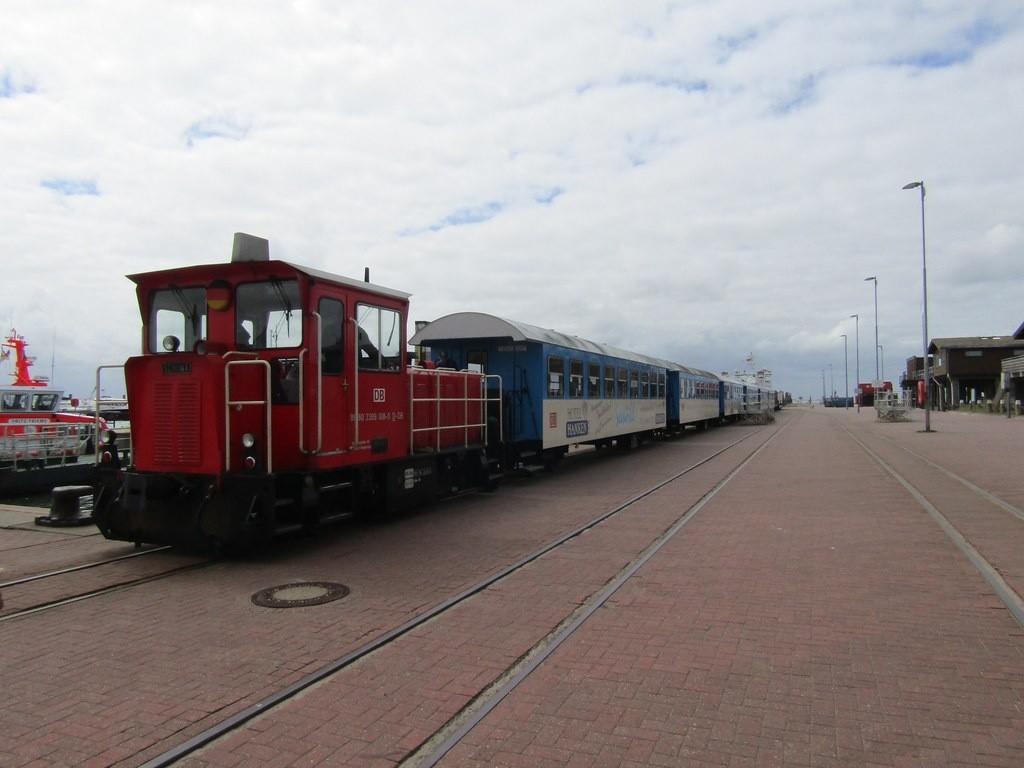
[92,260,784,554]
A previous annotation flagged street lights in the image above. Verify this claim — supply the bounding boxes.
[902,180,930,430]
[820,368,825,397]
[878,345,884,380]
[827,362,834,396]
[850,314,860,412]
[841,334,848,410]
[865,276,879,380]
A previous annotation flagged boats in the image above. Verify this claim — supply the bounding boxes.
[0,328,109,458]
[94,387,128,404]
[87,408,131,429]
